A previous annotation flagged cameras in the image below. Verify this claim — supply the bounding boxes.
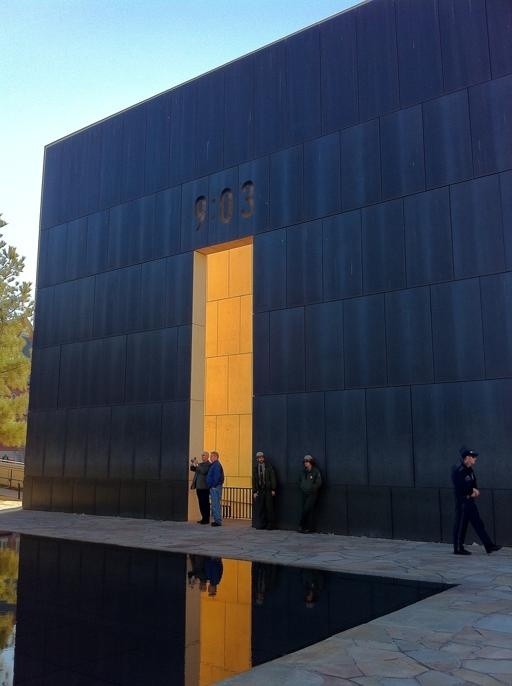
[191,457,196,463]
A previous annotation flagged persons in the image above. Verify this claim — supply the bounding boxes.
[190,451,212,525]
[252,452,276,530]
[187,553,223,596]
[1,453,8,463]
[254,562,268,605]
[300,570,318,609]
[293,454,322,534]
[206,451,223,526]
[451,449,503,555]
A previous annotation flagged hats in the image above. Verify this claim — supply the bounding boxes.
[256,452,264,458]
[461,448,480,459]
[304,455,313,461]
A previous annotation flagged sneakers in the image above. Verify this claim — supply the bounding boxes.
[453,545,504,556]
[197,519,222,527]
